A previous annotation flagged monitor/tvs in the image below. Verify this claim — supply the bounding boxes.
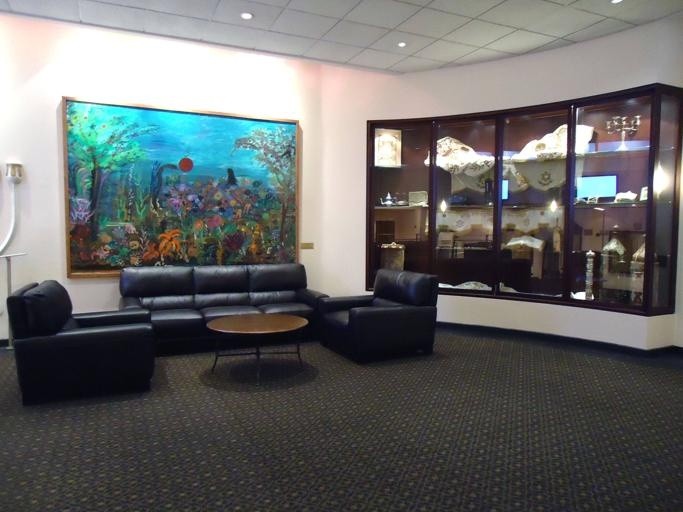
[576,174,618,199]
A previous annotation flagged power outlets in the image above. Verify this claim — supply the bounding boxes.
[302,243,314,249]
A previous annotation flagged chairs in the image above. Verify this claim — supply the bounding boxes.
[321,270,440,365]
[6,280,154,407]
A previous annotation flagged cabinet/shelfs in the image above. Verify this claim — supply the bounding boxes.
[368,83,676,316]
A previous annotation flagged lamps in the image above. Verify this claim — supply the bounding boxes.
[0,163,29,350]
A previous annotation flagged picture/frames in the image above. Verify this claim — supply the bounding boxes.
[61,96,301,278]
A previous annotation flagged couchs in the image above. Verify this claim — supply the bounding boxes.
[117,264,329,355]
